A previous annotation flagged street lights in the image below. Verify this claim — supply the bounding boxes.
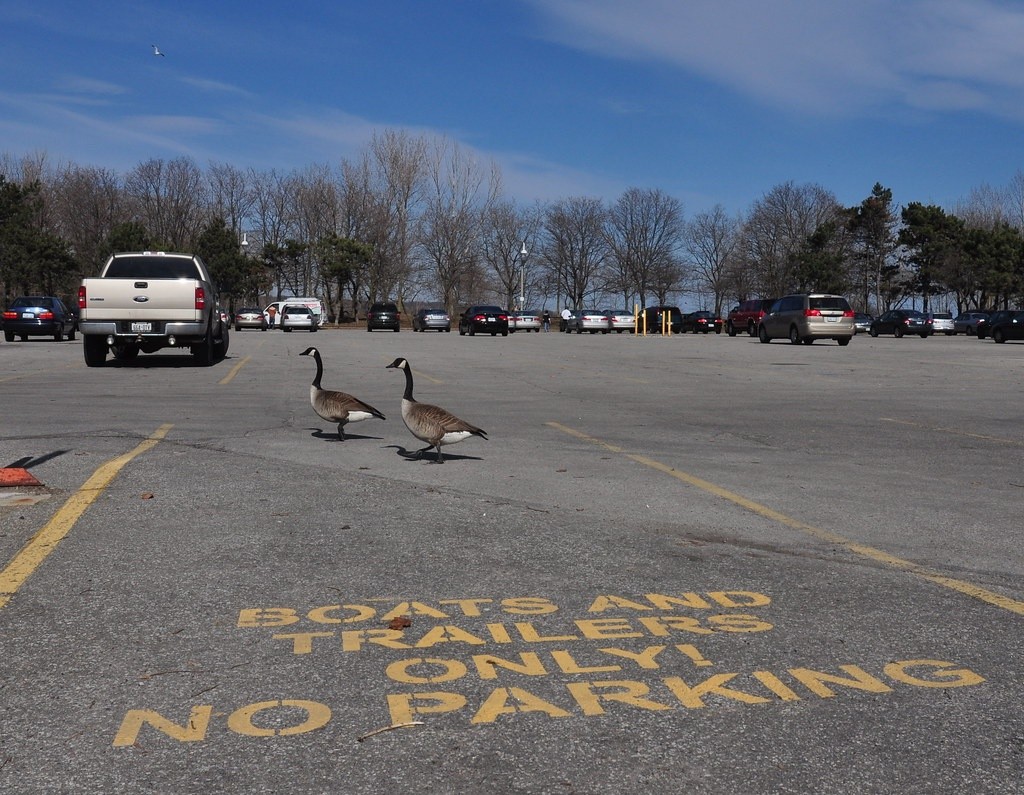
[520,242,527,311]
[241,233,248,308]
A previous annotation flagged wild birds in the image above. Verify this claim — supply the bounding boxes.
[386,358,489,464]
[299,347,386,442]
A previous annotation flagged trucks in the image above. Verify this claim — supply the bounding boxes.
[263,297,327,327]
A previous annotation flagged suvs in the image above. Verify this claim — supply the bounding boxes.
[727,299,780,338]
[758,292,855,346]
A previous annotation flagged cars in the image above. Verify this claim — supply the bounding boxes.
[412,308,451,332]
[459,306,509,337]
[681,311,723,334]
[507,310,541,333]
[220,309,232,329]
[854,309,1024,344]
[277,304,306,330]
[283,307,318,333]
[566,309,610,334]
[0,296,77,342]
[234,307,268,331]
[601,309,635,334]
[638,306,682,334]
[367,302,402,332]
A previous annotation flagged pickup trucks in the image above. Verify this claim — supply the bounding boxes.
[78,250,229,367]
